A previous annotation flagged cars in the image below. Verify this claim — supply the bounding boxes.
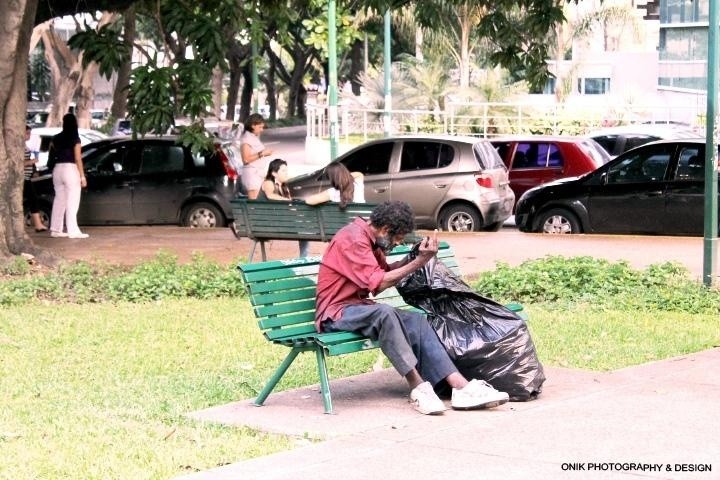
[587,128,706,159]
[22,138,248,227]
[27,128,127,172]
[491,138,612,225]
[515,139,720,237]
[281,137,515,232]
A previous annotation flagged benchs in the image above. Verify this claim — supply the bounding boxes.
[228,196,423,265]
[236,240,530,415]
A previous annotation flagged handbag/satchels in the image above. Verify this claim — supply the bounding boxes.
[47,146,56,170]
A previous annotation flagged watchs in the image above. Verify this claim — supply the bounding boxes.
[258,152,263,158]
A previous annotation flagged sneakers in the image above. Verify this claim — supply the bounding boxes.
[450,378,509,411]
[67,230,90,239]
[34,225,47,233]
[409,381,446,416]
[50,231,68,238]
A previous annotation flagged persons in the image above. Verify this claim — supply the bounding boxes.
[305,161,365,206]
[315,201,509,416]
[256,158,309,259]
[240,113,273,199]
[23,125,49,232]
[47,113,90,239]
